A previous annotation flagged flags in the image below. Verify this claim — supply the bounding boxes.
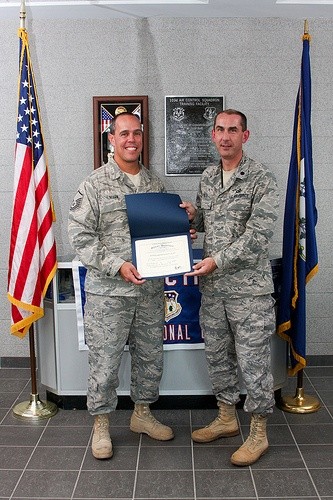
[6,28,57,339]
[278,39,318,376]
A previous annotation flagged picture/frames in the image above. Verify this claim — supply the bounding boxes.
[91,94,150,173]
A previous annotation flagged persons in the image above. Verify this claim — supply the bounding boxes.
[178,109,280,466]
[66,112,198,459]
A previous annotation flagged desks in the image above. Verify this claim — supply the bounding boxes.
[33,253,288,410]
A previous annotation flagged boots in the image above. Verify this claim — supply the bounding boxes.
[191,401,239,442]
[91,414,112,458]
[129,404,174,440]
[230,416,269,465]
